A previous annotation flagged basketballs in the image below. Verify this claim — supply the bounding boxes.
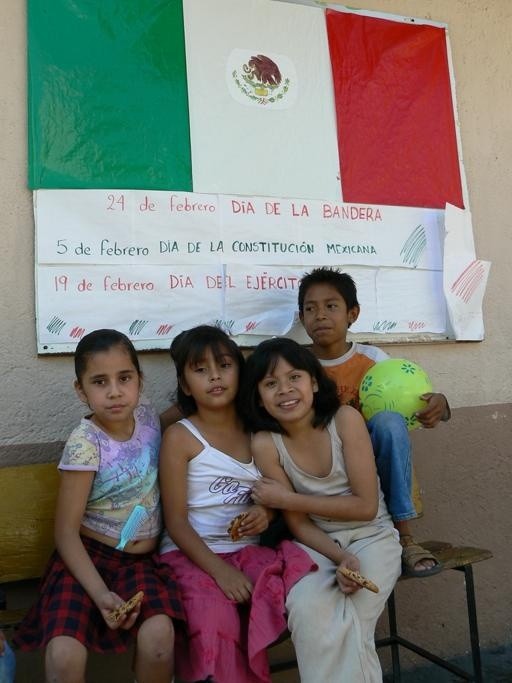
[359,358,434,432]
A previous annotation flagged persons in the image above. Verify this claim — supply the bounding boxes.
[0,623,18,683]
[233,335,404,682]
[295,266,453,580]
[158,322,317,681]
[20,328,194,682]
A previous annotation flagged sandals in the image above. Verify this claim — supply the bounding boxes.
[398,531,443,578]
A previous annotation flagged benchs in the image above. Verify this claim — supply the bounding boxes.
[0,461,493,683]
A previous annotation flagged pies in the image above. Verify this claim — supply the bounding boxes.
[338,565,379,592]
[228,513,248,542]
[105,590,144,623]
